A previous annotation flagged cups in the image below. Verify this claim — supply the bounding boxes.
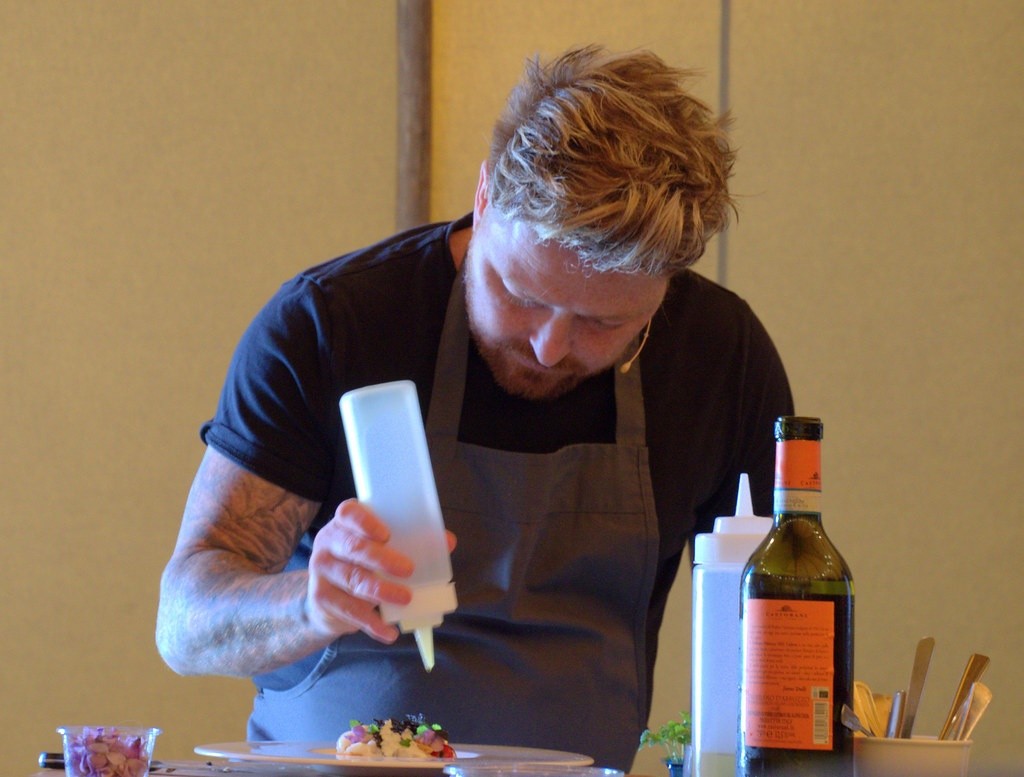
[853,735,973,777]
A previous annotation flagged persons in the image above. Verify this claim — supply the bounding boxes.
[155,55,794,773]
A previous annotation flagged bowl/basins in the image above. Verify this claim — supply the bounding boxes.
[56,724,163,777]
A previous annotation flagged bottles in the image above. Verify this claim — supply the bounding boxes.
[338,379,460,673]
[687,471,773,777]
[741,413,855,777]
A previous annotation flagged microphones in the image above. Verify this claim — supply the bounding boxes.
[619,318,652,374]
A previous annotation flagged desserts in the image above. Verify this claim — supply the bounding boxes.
[335,712,457,760]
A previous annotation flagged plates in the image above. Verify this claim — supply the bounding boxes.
[194,738,594,772]
